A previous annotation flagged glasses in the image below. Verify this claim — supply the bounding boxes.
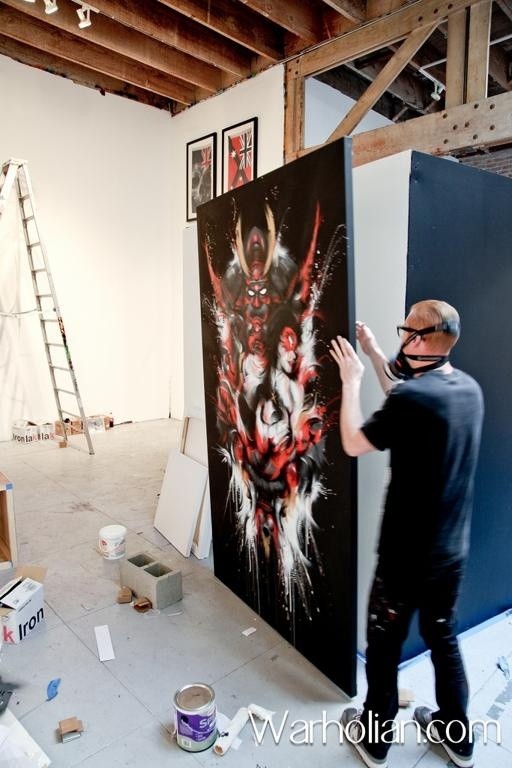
[397,326,415,336]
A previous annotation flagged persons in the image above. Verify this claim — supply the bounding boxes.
[329,300,485,768]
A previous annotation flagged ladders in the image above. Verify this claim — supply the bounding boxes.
[0,159,95,455]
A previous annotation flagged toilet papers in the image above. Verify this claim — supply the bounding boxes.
[214,707,250,755]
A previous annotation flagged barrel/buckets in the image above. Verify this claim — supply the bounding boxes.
[176,683,217,751]
[99,524,126,559]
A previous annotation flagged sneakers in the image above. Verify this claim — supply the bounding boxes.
[341,708,387,768]
[413,706,473,768]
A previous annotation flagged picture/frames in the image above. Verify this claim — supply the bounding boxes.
[186,117,257,222]
